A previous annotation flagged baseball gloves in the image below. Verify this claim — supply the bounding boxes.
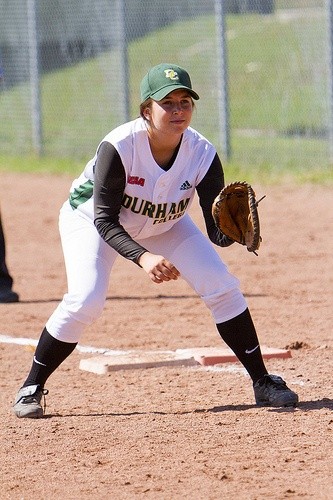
[212,182,261,252]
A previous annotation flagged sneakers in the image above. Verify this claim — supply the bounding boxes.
[14,386,44,418]
[252,374,301,407]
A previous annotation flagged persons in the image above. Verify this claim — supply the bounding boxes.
[13,62,296,419]
[0,217,18,302]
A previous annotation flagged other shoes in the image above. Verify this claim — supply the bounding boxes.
[0,288,19,303]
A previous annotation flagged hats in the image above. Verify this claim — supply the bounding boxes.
[140,63,200,102]
